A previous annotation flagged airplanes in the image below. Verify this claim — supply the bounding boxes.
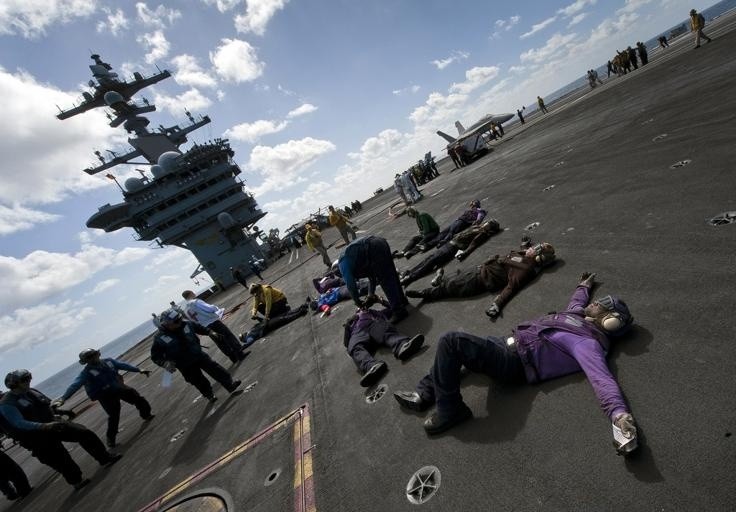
[436,110,515,162]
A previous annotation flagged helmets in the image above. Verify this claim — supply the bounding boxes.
[605,293,633,339]
[485,218,500,233]
[77,348,102,366]
[3,367,32,391]
[405,204,418,218]
[469,199,480,207]
[157,308,183,331]
[537,241,557,268]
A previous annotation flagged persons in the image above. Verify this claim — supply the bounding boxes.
[150,309,241,402]
[0,369,124,490]
[584,36,669,88]
[0,388,77,421]
[690,8,711,49]
[49,348,156,450]
[182,290,252,363]
[152,313,161,328]
[170,301,189,320]
[392,270,640,454]
[0,440,35,500]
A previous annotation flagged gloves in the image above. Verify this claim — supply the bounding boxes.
[208,328,226,343]
[44,421,68,433]
[484,303,500,319]
[457,253,467,262]
[49,396,65,408]
[576,269,597,289]
[162,358,178,374]
[610,411,641,456]
[139,366,154,378]
[521,236,531,247]
[57,407,78,420]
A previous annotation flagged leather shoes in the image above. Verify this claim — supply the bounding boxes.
[434,420,440,420]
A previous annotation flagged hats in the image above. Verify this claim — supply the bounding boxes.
[689,9,698,15]
[328,206,334,211]
[394,173,402,179]
[304,223,313,230]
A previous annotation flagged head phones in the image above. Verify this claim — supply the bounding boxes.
[596,295,624,332]
[79,348,101,365]
[7,370,15,388]
[534,241,546,263]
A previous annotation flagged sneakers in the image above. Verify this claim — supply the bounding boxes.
[231,349,252,366]
[394,331,427,362]
[72,477,92,493]
[106,440,121,449]
[385,296,410,324]
[390,384,426,414]
[419,409,474,439]
[395,266,411,287]
[430,266,445,287]
[205,391,219,404]
[391,242,447,259]
[405,288,425,298]
[144,414,156,423]
[227,377,242,394]
[358,357,388,388]
[102,453,124,470]
[693,39,713,50]
